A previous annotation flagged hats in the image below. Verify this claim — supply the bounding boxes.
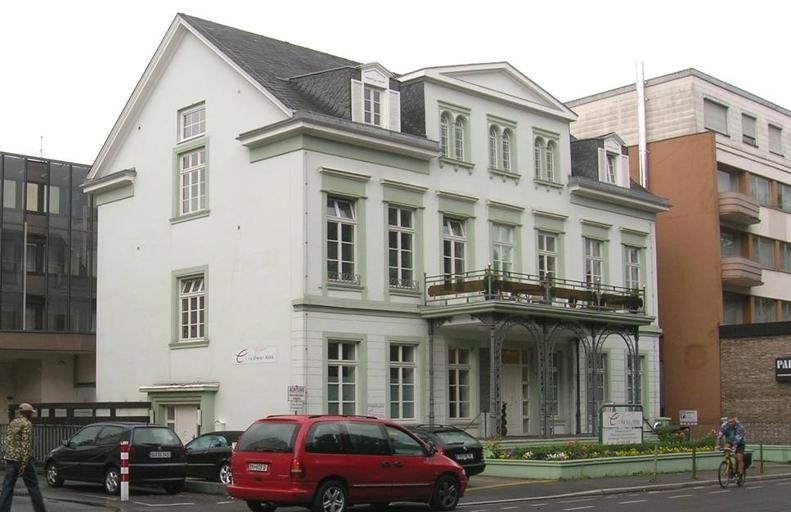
[20,402,37,413]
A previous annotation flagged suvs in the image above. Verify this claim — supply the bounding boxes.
[45,421,188,493]
[227,414,470,511]
[403,424,488,476]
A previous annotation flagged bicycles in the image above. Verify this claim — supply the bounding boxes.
[717,446,753,488]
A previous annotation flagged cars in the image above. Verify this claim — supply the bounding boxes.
[185,430,245,485]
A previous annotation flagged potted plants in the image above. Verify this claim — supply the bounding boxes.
[479,266,501,302]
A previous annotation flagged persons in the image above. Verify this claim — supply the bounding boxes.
[715,416,746,483]
[0,402,47,512]
